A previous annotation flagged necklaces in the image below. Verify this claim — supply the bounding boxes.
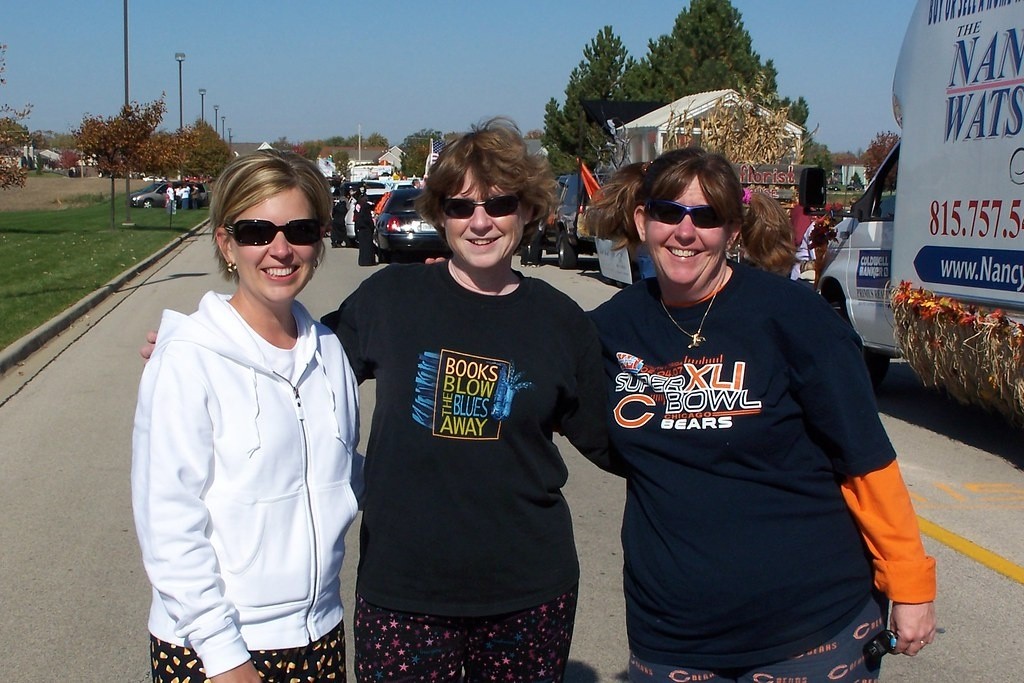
[660,286,717,348]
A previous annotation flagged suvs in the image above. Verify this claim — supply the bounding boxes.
[528,172,623,271]
[127,179,208,210]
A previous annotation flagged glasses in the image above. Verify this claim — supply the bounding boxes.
[642,199,728,228]
[864,627,898,659]
[440,188,524,219]
[224,213,321,246]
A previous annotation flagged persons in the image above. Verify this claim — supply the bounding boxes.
[140,126,626,683]
[587,149,936,682]
[412,180,420,188]
[795,209,836,267]
[331,187,379,266]
[166,183,198,214]
[130,148,368,683]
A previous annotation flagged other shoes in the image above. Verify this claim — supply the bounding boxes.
[331,242,343,248]
[532,264,540,268]
[521,263,530,267]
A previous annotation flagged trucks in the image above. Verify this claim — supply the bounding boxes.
[798,0,1024,392]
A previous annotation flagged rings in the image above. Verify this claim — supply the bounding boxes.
[921,641,926,645]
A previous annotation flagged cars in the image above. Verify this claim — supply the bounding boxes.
[325,176,429,247]
[367,188,454,264]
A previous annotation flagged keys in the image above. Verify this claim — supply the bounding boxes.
[863,630,899,662]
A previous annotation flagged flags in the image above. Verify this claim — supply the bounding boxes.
[578,159,604,203]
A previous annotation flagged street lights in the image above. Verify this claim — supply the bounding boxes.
[213,105,219,132]
[199,88,206,122]
[174,52,186,128]
[221,116,225,140]
